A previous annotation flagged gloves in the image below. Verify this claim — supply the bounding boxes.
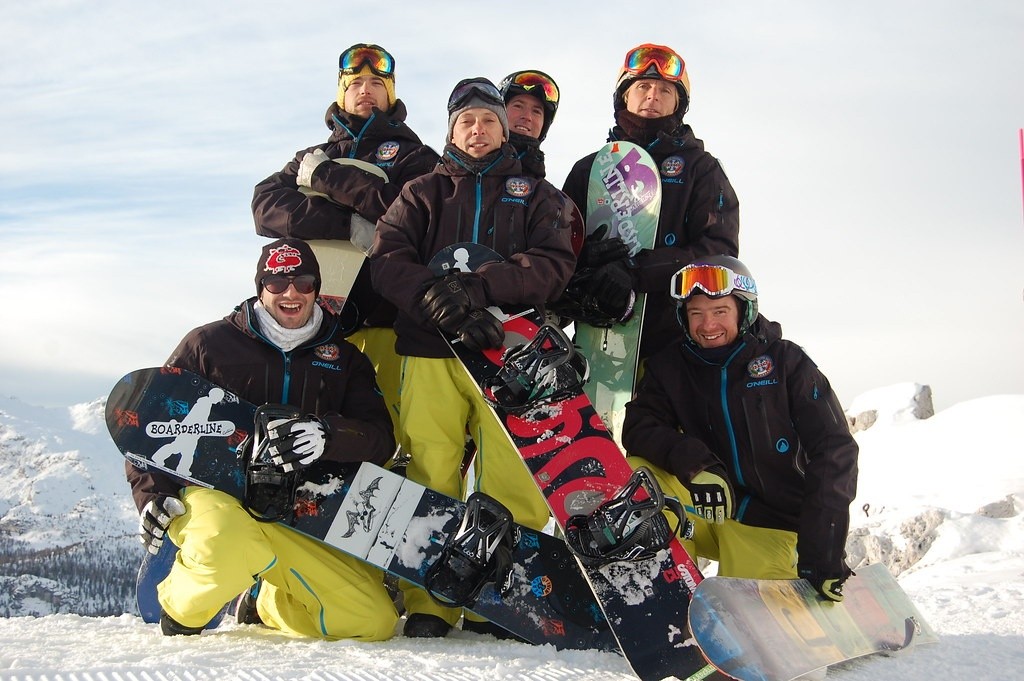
[572,222,630,279]
[295,147,330,187]
[449,308,506,353]
[420,269,494,324]
[267,415,325,473]
[690,464,735,525]
[588,259,643,312]
[139,496,186,555]
[351,212,377,256]
[812,575,845,602]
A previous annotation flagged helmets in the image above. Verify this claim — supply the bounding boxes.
[676,254,759,339]
[496,71,557,140]
[615,43,690,114]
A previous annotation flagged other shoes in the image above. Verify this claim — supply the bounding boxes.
[234,586,263,625]
[403,613,449,638]
[160,609,205,635]
[474,621,525,642]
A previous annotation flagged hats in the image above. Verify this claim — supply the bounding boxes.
[255,237,321,299]
[448,77,509,143]
[337,43,396,110]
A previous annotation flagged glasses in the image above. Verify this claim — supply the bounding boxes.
[625,45,685,80]
[510,70,560,111]
[260,274,317,294]
[447,80,505,112]
[670,263,736,303]
[338,43,394,77]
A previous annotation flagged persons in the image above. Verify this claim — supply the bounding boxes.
[622,256,860,602]
[125,238,399,642]
[366,77,577,645]
[497,70,560,179]
[251,43,441,619]
[562,44,739,458]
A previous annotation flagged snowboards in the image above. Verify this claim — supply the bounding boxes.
[105,365,619,652]
[295,158,389,314]
[424,240,737,681]
[687,562,917,681]
[553,140,663,540]
